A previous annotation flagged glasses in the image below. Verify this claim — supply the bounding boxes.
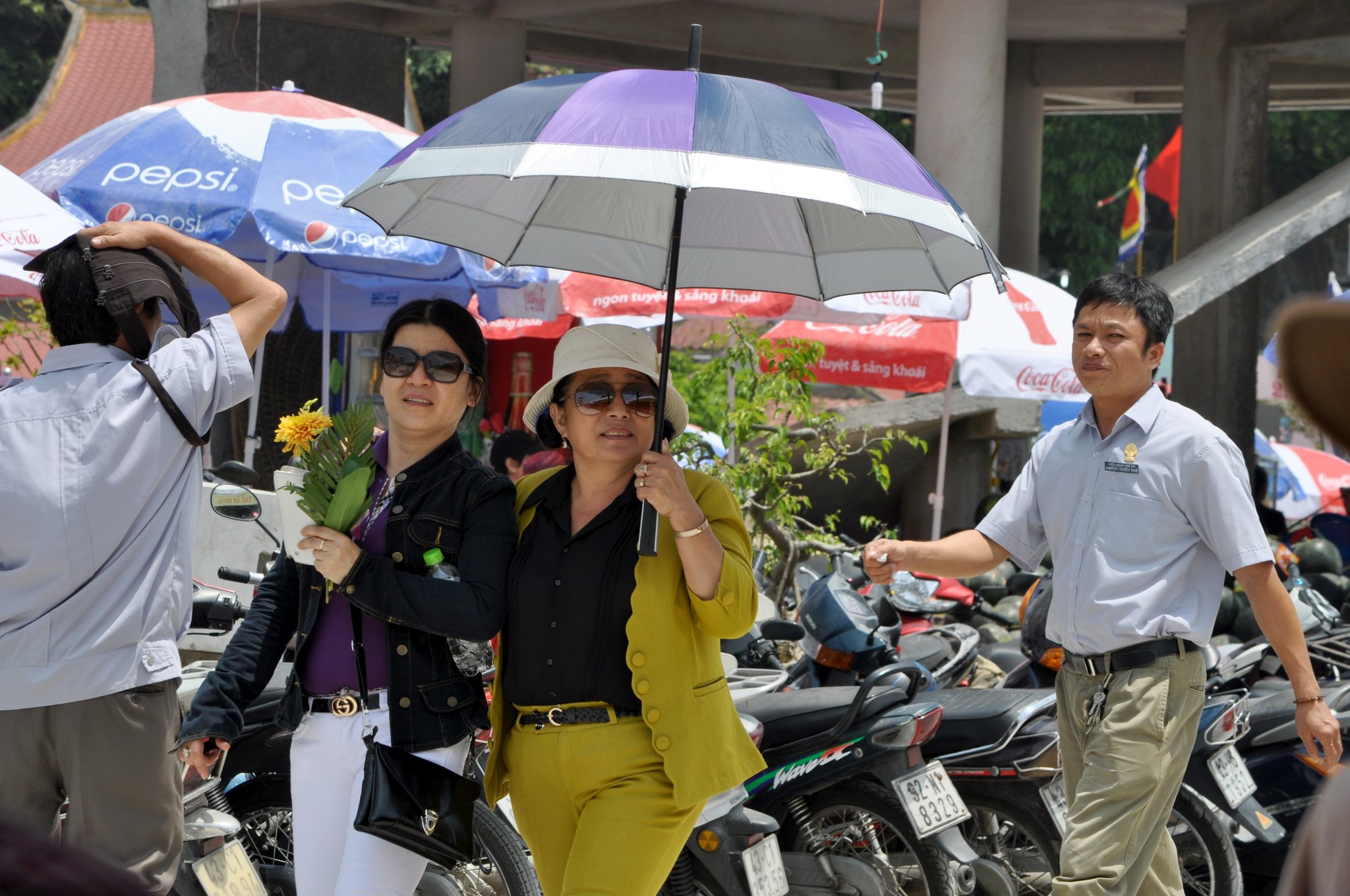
[560,381,659,418]
[381,345,475,384]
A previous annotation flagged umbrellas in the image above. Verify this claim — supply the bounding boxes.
[15,77,564,480]
[1,162,90,305]
[463,267,1096,409]
[343,22,1012,564]
[1251,332,1350,525]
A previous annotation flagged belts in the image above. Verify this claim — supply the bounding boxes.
[515,705,640,727]
[303,691,389,717]
[1064,637,1203,676]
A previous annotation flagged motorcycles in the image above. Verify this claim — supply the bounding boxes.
[173,461,1350,896]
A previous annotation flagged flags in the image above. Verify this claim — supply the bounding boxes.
[1112,143,1148,259]
[1144,126,1183,216]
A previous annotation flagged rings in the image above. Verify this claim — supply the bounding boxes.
[639,476,645,487]
[319,538,326,551]
[640,463,650,477]
[182,747,191,760]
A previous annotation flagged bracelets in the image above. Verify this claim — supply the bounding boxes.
[672,517,709,538]
[1293,693,1326,705]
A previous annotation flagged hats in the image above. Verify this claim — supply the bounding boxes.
[1276,299,1350,457]
[523,324,689,444]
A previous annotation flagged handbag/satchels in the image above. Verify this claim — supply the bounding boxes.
[23,233,211,448]
[354,726,482,871]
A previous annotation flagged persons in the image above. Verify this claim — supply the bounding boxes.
[862,272,1344,894]
[171,296,519,896]
[484,318,772,896]
[1,218,286,894]
